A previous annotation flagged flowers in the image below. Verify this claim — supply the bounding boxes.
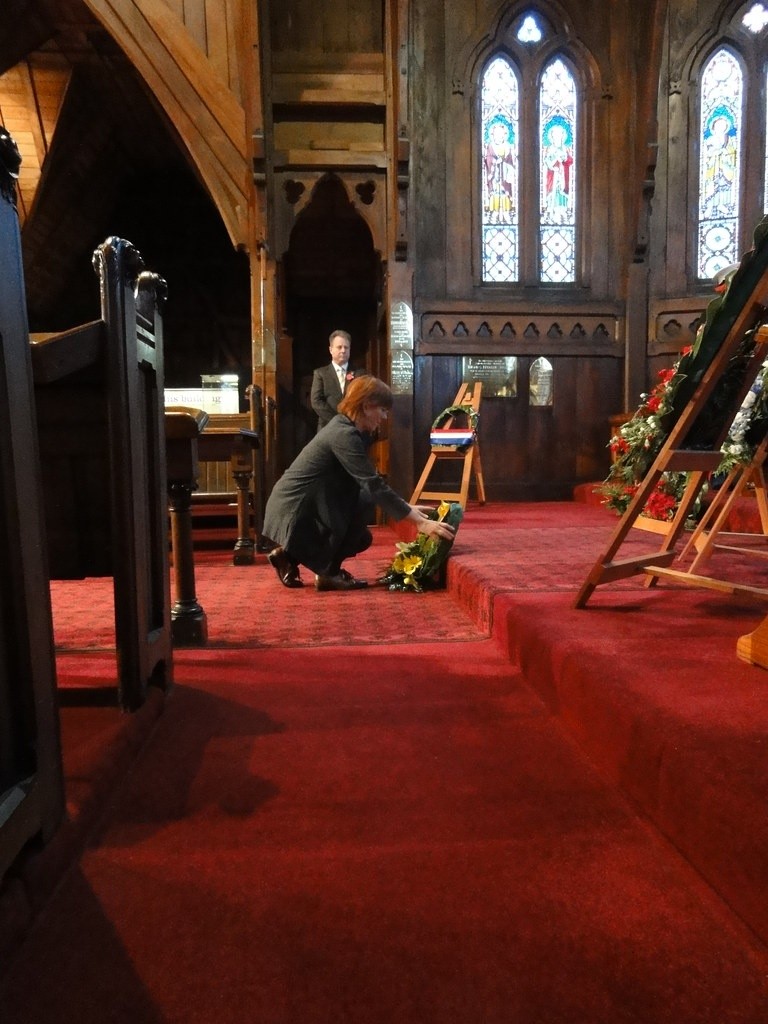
[592,280,768,526]
[376,499,462,593]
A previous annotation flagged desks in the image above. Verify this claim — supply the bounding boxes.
[164,406,209,646]
[196,426,267,564]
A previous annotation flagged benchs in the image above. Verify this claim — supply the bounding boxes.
[191,385,273,553]
[29,237,172,718]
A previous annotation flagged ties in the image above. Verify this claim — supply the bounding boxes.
[338,367,346,395]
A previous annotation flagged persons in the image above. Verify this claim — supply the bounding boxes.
[309,329,366,434]
[259,374,455,592]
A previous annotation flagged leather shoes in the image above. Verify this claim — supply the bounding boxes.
[269,549,304,589]
[317,569,367,590]
